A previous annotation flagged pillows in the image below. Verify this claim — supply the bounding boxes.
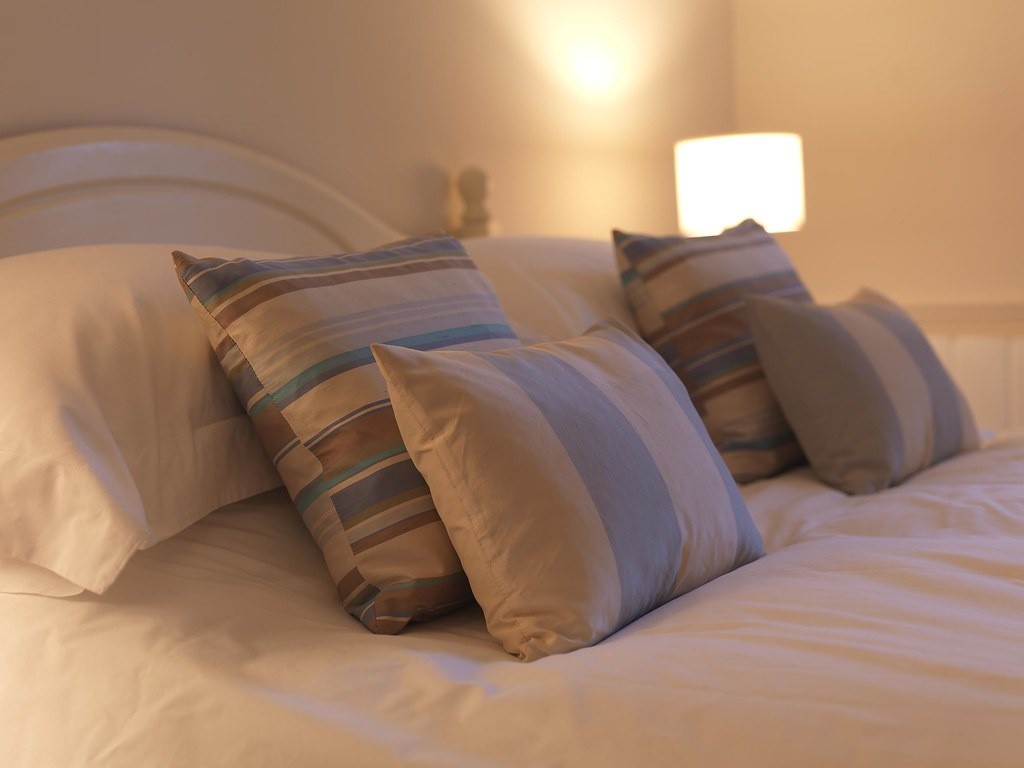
[0,241,301,599]
[454,235,618,345]
[170,232,562,631]
[370,316,765,663]
[611,218,817,487]
[747,288,981,495]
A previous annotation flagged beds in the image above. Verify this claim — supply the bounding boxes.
[0,127,1024,768]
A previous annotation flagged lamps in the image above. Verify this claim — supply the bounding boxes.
[673,131,807,238]
